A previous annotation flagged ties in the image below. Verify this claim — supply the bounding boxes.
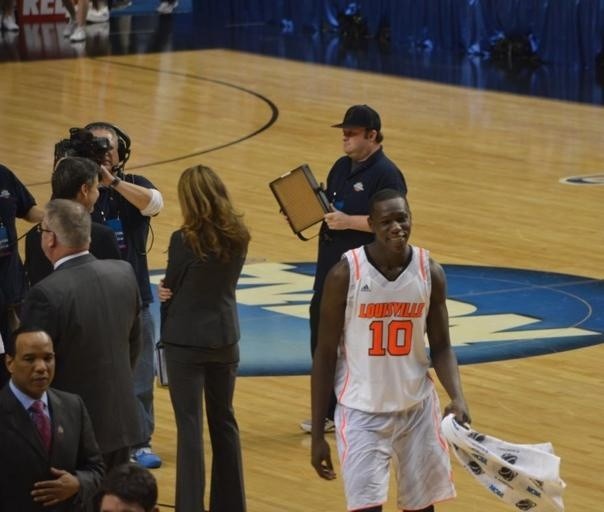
[30,400,52,451]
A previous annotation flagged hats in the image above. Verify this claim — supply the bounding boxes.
[331,105,380,131]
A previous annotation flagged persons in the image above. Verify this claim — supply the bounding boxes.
[311,188,471,512]
[0,123,164,511]
[158,164,250,512]
[0,0,179,41]
[280,104,408,434]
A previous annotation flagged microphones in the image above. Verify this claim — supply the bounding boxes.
[111,152,131,172]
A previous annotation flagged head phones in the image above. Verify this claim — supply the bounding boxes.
[72,123,126,164]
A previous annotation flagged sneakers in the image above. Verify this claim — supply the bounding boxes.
[301,416,335,433]
[64,19,86,42]
[158,1,180,15]
[128,447,161,468]
[3,14,21,31]
